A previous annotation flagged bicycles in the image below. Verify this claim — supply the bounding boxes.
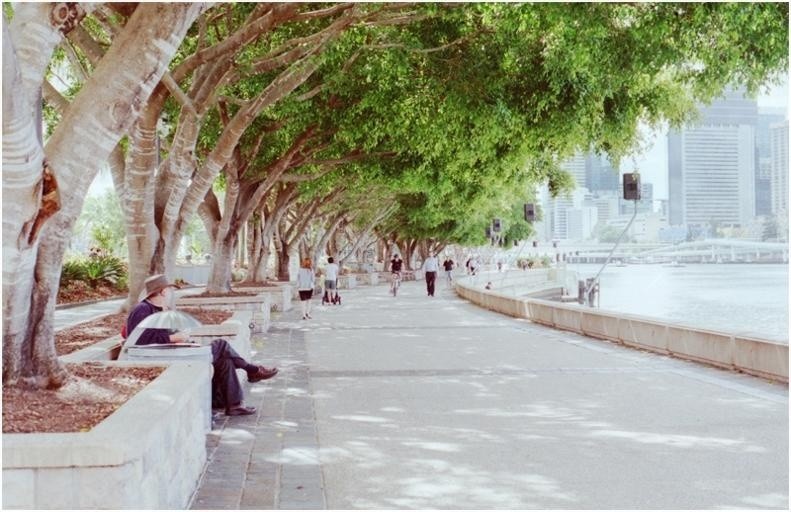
[389,271,401,298]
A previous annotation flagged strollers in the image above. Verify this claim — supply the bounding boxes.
[322,290,341,306]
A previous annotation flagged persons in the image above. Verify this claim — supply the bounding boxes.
[295,257,317,323]
[443,255,457,288]
[466,257,473,276]
[416,255,425,282]
[485,282,493,290]
[421,251,441,299]
[126,273,279,419]
[388,253,406,294]
[320,257,340,307]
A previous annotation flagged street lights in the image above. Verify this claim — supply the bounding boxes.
[585,173,641,307]
[469,204,537,292]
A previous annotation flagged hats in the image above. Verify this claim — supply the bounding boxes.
[137,274,181,303]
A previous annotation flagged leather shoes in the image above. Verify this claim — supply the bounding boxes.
[248,366,279,383]
[225,404,257,417]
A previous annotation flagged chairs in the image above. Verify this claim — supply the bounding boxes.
[116,310,237,361]
